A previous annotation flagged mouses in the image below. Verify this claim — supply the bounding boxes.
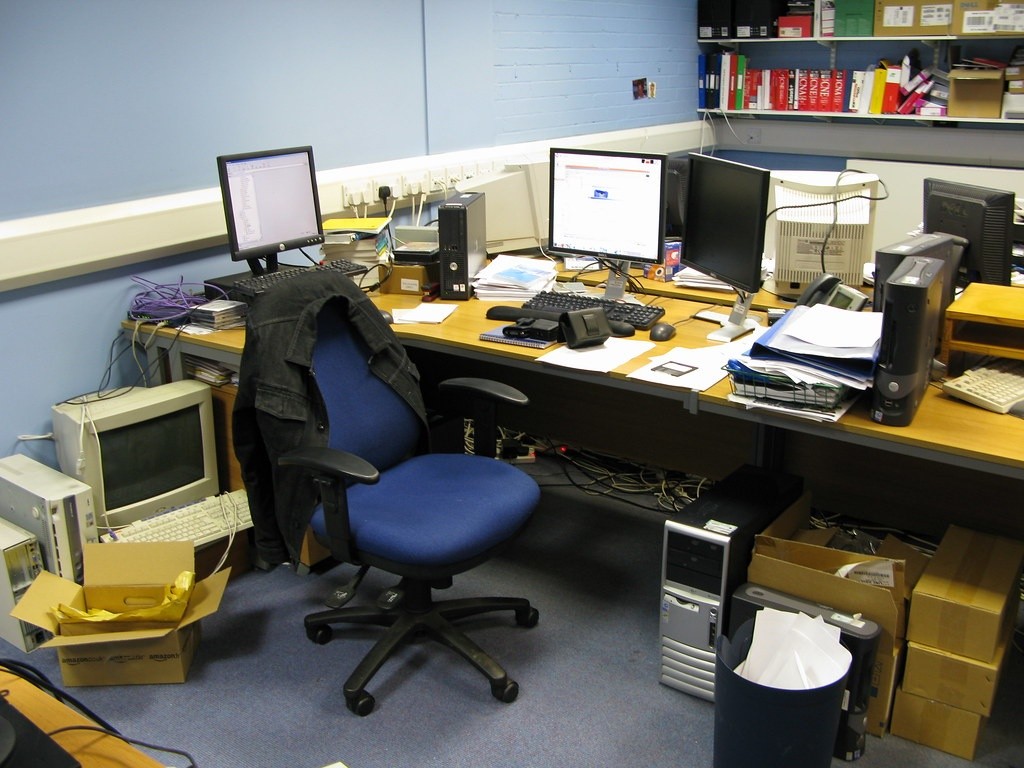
[379,310,394,324]
[649,322,676,341]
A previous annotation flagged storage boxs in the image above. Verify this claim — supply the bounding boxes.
[8,543,232,687]
[836,0,1024,118]
[747,492,1024,760]
[777,16,813,38]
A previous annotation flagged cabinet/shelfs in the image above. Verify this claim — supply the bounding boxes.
[697,31,1024,125]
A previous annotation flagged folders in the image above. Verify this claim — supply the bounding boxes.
[750,309,878,390]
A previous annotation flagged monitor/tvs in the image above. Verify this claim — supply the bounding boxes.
[925,178,1014,288]
[454,157,553,260]
[51,380,220,538]
[678,152,770,342]
[765,169,879,301]
[216,144,325,274]
[550,147,668,309]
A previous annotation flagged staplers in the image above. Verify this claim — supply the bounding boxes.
[420,282,440,302]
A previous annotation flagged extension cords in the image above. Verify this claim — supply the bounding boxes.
[493,444,536,464]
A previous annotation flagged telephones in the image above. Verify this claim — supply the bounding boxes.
[793,271,869,312]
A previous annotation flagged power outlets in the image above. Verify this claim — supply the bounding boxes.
[746,128,761,144]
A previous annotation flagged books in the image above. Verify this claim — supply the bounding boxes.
[472,253,559,301]
[697,53,865,114]
[322,217,392,288]
[1008,45,1024,65]
[184,355,237,387]
[952,57,1008,69]
[479,325,558,350]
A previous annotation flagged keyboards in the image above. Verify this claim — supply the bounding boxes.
[233,259,368,297]
[99,488,253,549]
[941,360,1024,416]
[521,292,666,331]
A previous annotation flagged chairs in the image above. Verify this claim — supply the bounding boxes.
[245,270,539,715]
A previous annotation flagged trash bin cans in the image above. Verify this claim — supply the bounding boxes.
[714,608,853,768]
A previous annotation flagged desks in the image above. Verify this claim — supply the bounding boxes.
[121,242,1024,579]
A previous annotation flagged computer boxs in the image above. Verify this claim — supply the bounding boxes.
[659,463,804,705]
[438,192,487,301]
[867,234,956,428]
[204,264,306,303]
[727,583,881,762]
[0,453,100,654]
